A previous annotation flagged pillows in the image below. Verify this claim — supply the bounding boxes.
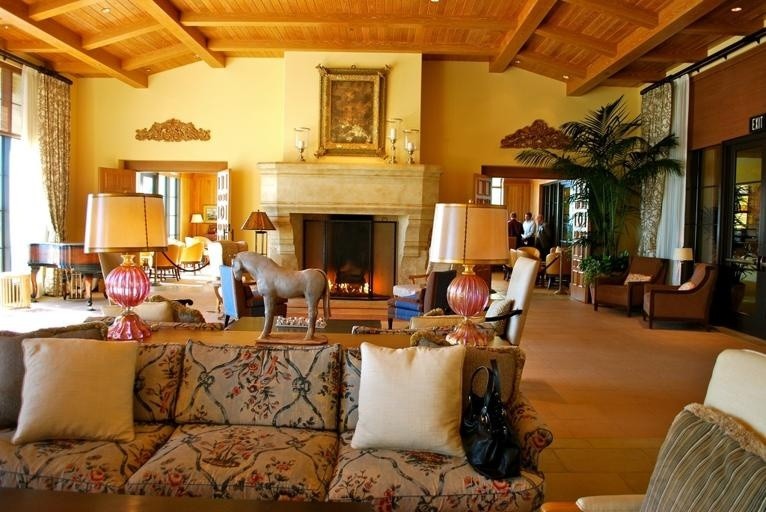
[343,347,524,447]
[132,343,184,424]
[176,339,341,430]
[351,342,466,460]
[103,302,173,324]
[12,337,139,446]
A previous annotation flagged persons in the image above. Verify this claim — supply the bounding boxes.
[507,212,525,247]
[534,214,553,260]
[521,213,538,245]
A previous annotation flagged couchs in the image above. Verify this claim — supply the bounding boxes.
[0,331,553,512]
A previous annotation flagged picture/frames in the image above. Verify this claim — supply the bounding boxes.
[203,205,217,223]
[314,64,392,160]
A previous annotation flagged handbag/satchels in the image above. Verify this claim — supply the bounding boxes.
[459,355,524,482]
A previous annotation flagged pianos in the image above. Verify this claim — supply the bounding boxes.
[28,243,107,311]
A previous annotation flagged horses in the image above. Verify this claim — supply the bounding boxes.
[232,251,331,340]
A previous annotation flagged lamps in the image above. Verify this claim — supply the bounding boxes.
[294,127,310,162]
[386,118,403,164]
[191,214,203,236]
[84,190,168,342]
[403,129,419,165]
[672,248,693,286]
[240,209,276,256]
[429,198,511,347]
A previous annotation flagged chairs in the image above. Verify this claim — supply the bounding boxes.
[387,270,457,329]
[409,257,540,348]
[140,236,210,281]
[643,263,716,333]
[541,349,766,512]
[504,247,571,290]
[594,255,669,318]
[217,265,289,326]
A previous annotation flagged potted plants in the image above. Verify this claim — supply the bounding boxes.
[513,93,684,304]
[729,185,756,310]
